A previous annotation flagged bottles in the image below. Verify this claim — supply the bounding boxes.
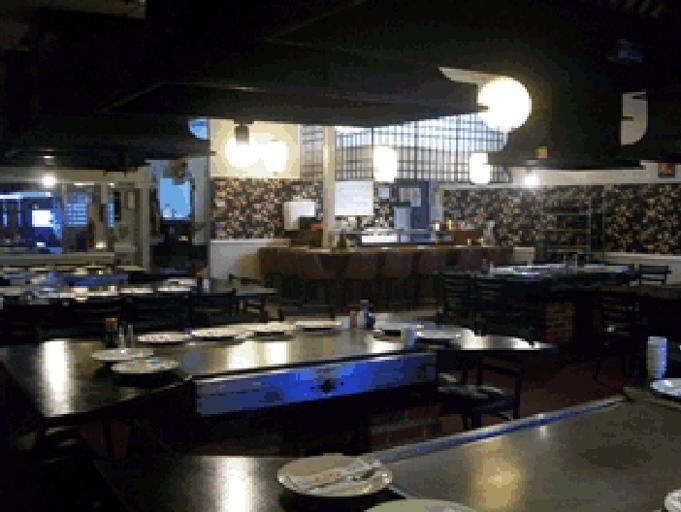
[104,318,120,348]
[357,300,369,330]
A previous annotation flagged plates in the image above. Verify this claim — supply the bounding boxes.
[362,494,476,512]
[414,327,465,343]
[488,262,629,279]
[111,356,179,376]
[649,373,681,400]
[271,451,395,500]
[295,319,343,332]
[191,328,237,339]
[246,322,291,336]
[375,319,422,334]
[91,347,154,362]
[136,331,193,345]
